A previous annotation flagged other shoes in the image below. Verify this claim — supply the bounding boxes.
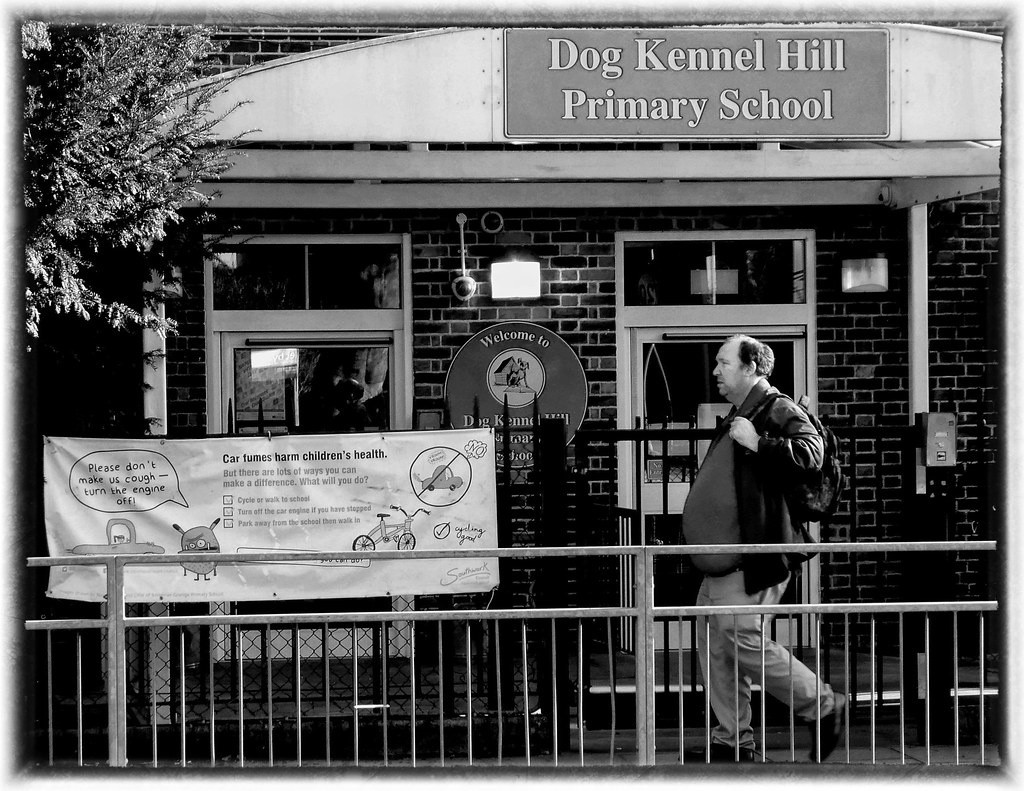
[678,742,753,763]
[806,691,846,762]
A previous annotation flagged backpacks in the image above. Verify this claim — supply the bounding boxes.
[762,391,844,519]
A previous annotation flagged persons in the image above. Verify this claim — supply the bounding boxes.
[677,333,850,762]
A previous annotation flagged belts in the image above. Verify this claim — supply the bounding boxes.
[704,562,743,578]
